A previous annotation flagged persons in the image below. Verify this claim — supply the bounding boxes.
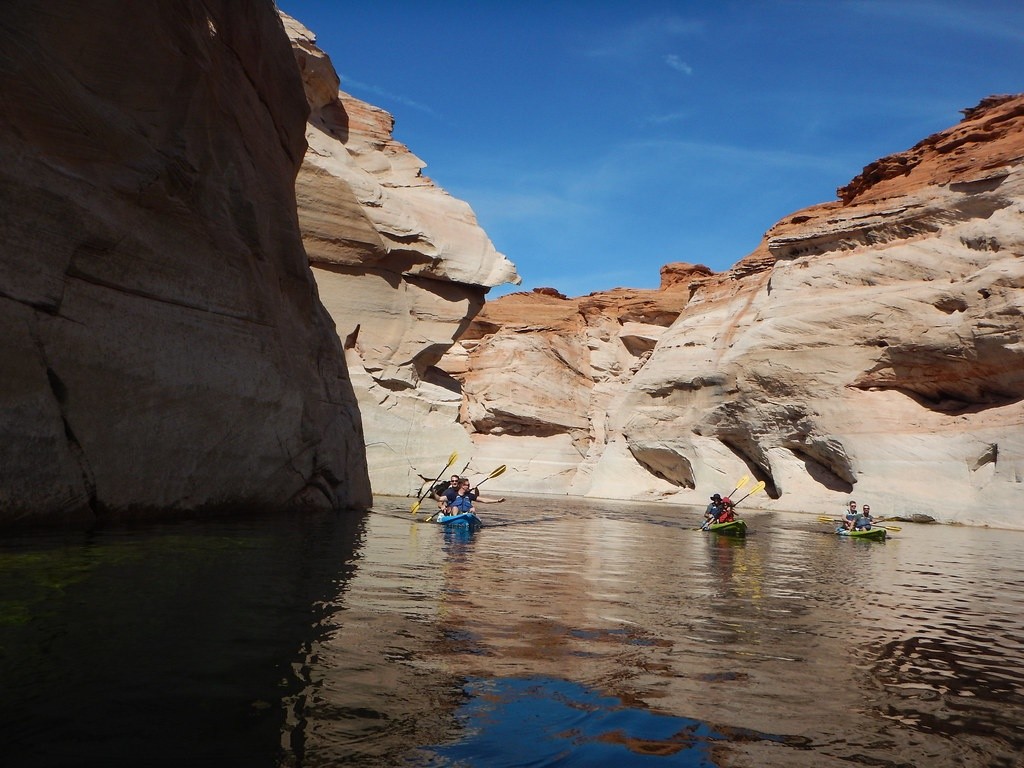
[846,504,885,532]
[429,479,506,514]
[437,475,468,514]
[704,494,722,520]
[711,497,739,524]
[841,501,860,530]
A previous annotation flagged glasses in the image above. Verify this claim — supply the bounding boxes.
[463,483,470,486]
[864,508,869,509]
[851,505,856,508]
[450,480,458,482]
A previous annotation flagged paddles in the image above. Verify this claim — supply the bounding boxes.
[700,475,766,532]
[817,516,902,532]
[410,451,458,513]
[423,464,506,521]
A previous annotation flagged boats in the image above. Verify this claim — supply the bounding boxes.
[701,518,748,536]
[835,525,888,542]
[436,510,482,529]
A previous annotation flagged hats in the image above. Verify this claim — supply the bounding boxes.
[710,494,723,503]
[720,496,731,505]
